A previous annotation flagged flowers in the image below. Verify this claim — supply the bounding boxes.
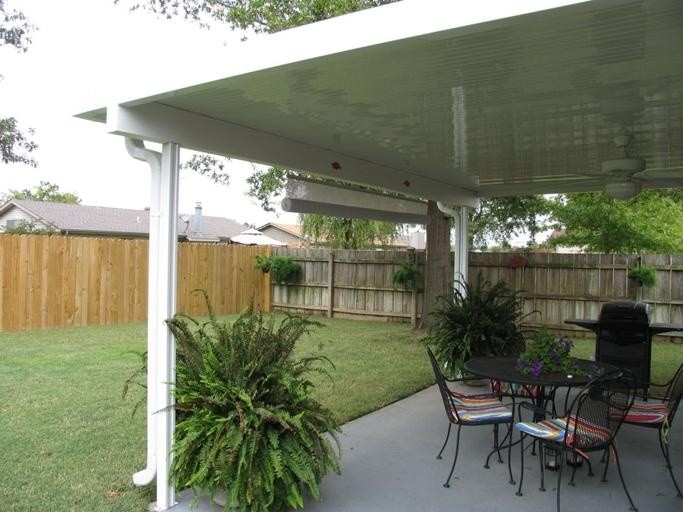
[517,331,577,377]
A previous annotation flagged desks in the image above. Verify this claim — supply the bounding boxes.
[565,300,682,402]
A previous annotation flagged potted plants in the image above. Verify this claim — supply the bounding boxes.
[419,270,542,388]
[121,284,349,512]
